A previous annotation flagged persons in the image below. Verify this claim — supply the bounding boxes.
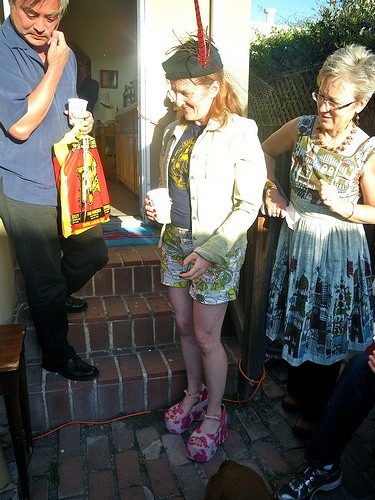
[0,0,109,379]
[262,45,375,500]
[144,40,268,462]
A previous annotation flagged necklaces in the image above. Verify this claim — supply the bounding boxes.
[316,119,359,154]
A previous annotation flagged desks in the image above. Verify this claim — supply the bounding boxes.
[96,125,114,174]
[0,324,35,500]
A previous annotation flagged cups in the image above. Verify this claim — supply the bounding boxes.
[68,97,88,126]
[147,188,171,224]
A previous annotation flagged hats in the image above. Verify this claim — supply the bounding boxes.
[162,0,223,79]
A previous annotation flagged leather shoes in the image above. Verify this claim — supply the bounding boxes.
[66,296,88,313]
[42,346,99,381]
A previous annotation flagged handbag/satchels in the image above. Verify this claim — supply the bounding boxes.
[52,121,110,238]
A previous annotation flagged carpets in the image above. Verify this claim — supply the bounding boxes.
[100,225,159,245]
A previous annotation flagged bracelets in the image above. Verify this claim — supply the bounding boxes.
[346,202,355,220]
[264,181,277,194]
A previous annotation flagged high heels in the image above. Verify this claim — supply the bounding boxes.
[164,383,209,434]
[186,403,228,462]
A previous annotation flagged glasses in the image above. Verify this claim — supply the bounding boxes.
[312,88,357,113]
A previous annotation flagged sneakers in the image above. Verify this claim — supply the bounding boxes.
[273,463,342,500]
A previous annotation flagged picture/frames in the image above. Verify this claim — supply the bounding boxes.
[101,69,120,89]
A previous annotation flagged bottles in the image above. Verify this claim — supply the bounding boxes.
[124,86,131,107]
[130,81,135,104]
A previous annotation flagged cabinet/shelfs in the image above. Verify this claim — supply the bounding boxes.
[116,106,140,192]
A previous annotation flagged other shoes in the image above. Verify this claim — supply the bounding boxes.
[293,425,310,439]
[281,401,296,413]
